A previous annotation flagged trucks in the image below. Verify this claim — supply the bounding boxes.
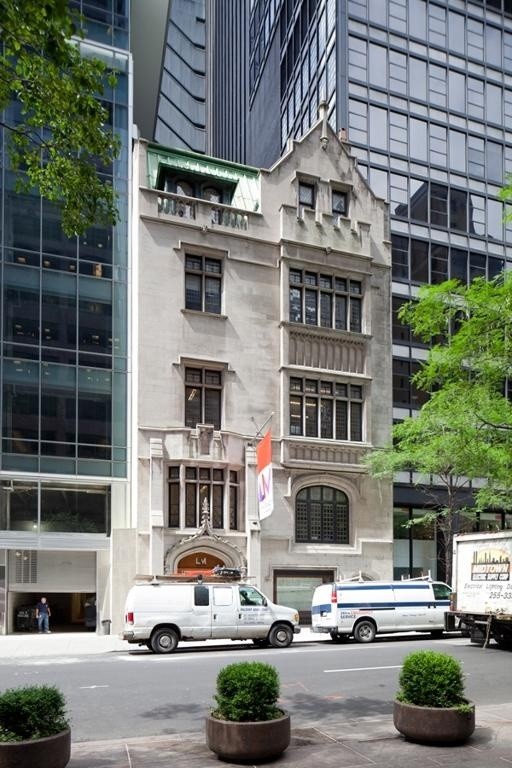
[444,528,512,650]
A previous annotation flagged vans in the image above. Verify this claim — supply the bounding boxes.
[309,568,466,644]
[121,563,303,656]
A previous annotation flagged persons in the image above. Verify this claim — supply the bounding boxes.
[35,596,52,634]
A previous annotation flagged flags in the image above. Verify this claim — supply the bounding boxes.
[256,425,274,522]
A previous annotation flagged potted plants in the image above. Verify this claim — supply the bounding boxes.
[391,649,476,739]
[0,683,72,768]
[202,661,291,760]
